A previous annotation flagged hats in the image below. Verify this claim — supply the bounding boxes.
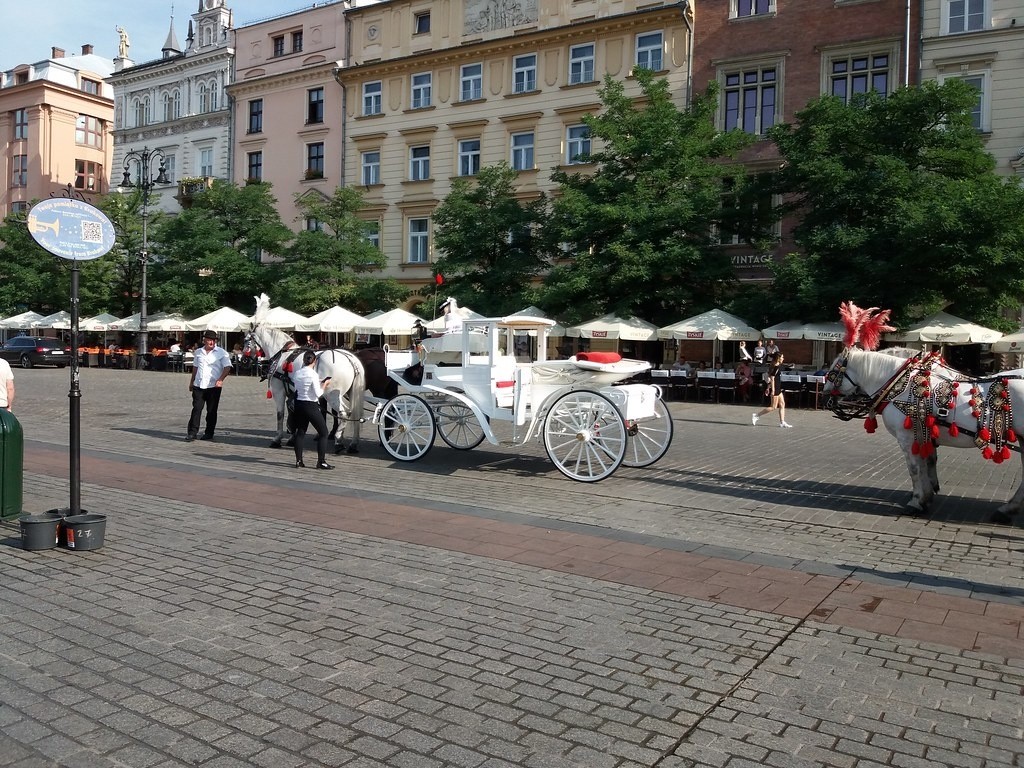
[201,330,219,340]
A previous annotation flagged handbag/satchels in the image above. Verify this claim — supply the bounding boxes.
[763,383,772,399]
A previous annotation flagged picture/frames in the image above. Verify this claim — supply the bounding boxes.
[389,335,397,345]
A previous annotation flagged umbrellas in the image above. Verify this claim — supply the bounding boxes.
[762,311,1024,372]
[657,309,761,372]
[0,306,566,358]
[566,311,659,353]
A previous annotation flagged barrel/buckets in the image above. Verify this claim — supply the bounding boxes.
[41,508,107,550]
[17,515,62,550]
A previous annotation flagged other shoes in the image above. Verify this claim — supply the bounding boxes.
[200,434,212,440]
[184,434,197,442]
[296,459,305,468]
[317,461,335,470]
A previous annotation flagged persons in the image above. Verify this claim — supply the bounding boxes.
[169,341,181,353]
[108,339,120,349]
[0,358,15,413]
[674,340,779,403]
[192,343,199,350]
[231,343,241,353]
[183,344,190,353]
[183,330,234,442]
[813,365,829,376]
[304,335,319,349]
[293,351,336,470]
[752,352,794,428]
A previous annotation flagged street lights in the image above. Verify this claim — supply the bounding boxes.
[118,147,173,355]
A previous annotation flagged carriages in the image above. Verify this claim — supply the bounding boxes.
[244,315,675,484]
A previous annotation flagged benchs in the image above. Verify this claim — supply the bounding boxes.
[470,356,531,387]
[421,333,488,353]
[532,352,621,386]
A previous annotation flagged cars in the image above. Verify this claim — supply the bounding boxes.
[0,336,71,370]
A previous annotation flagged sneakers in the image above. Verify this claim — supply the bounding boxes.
[752,413,759,425]
[780,421,792,428]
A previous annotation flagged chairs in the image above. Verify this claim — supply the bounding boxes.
[185,352,268,376]
[651,367,825,410]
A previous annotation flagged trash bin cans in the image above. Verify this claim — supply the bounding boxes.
[0,408,31,521]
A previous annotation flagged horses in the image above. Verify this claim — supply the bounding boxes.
[819,346,1024,521]
[242,322,396,454]
